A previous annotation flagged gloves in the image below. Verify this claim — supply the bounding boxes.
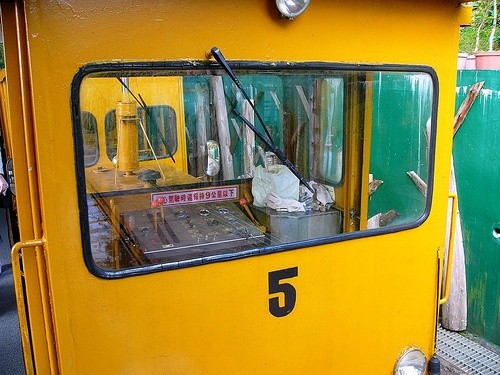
[264,194,306,212]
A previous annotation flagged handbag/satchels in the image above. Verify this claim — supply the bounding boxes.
[251,164,300,208]
